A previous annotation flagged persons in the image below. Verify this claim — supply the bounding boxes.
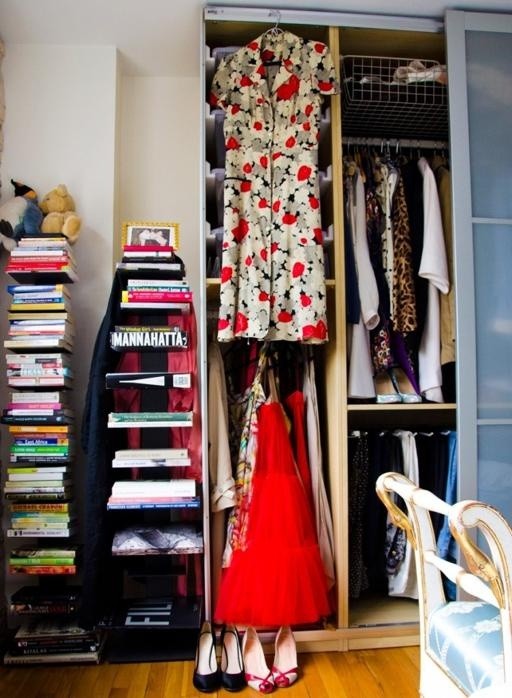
[132,229,169,246]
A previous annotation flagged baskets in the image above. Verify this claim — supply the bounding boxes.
[340,54,449,139]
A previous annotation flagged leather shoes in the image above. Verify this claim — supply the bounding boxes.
[241,624,275,693]
[391,365,423,403]
[272,623,300,688]
[220,623,245,692]
[373,371,402,404]
[192,622,221,692]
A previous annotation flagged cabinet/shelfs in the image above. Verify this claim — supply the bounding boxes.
[0,232,104,668]
[83,250,202,665]
[194,6,512,650]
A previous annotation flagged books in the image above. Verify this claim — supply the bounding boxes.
[107,480,200,511]
[111,325,189,351]
[117,244,191,314]
[0,231,105,671]
[110,594,203,630]
[111,524,203,555]
[107,412,193,427]
[105,372,193,390]
[112,448,191,468]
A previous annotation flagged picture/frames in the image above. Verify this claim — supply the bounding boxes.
[121,221,180,252]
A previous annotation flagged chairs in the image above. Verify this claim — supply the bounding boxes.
[374,472,511,698]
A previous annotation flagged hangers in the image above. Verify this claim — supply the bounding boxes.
[259,8,285,66]
[342,137,448,165]
[206,306,222,352]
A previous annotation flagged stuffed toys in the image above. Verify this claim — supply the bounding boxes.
[32,182,86,244]
[1,178,46,253]
